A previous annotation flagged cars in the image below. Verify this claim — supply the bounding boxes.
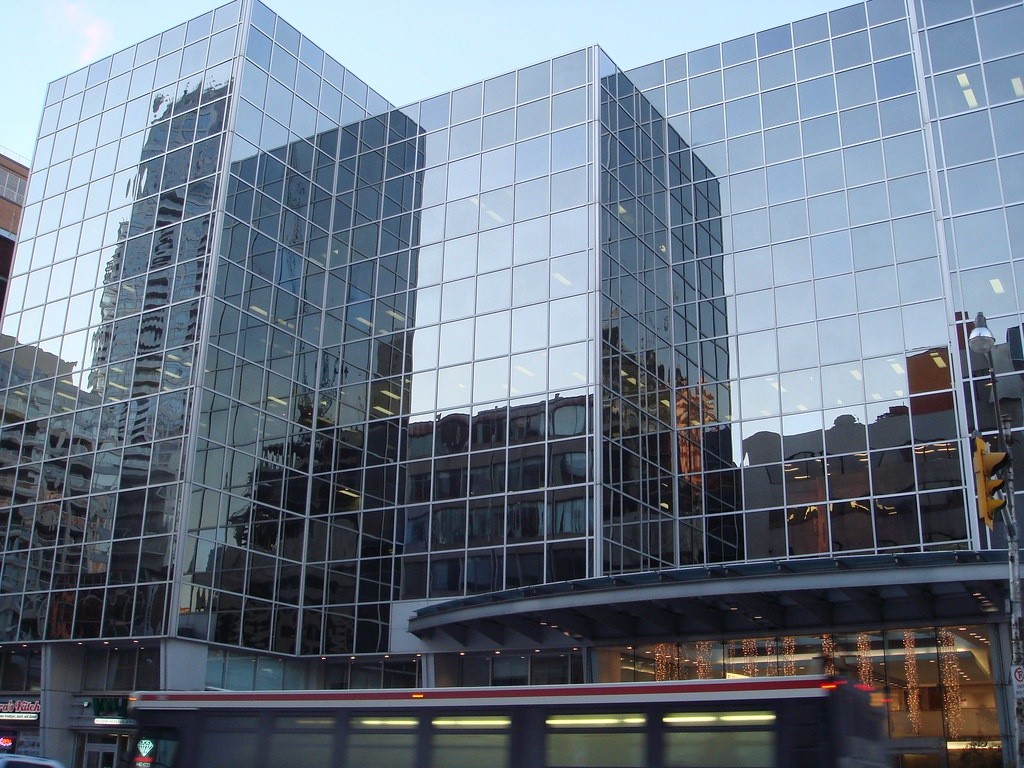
[0,752,65,768]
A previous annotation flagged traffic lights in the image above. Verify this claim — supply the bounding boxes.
[973,435,1008,531]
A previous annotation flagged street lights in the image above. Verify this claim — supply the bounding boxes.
[968,311,1024,768]
